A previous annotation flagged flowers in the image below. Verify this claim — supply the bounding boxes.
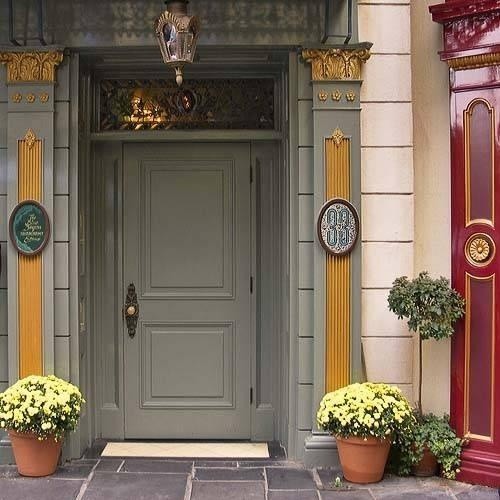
[0,372,86,441]
[314,377,416,446]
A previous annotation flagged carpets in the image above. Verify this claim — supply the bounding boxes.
[101,441,270,460]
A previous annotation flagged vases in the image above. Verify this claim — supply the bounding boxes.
[7,425,65,477]
[332,430,393,484]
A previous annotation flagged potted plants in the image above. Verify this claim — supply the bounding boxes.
[385,271,468,479]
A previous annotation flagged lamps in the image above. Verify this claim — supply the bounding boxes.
[153,0,201,89]
